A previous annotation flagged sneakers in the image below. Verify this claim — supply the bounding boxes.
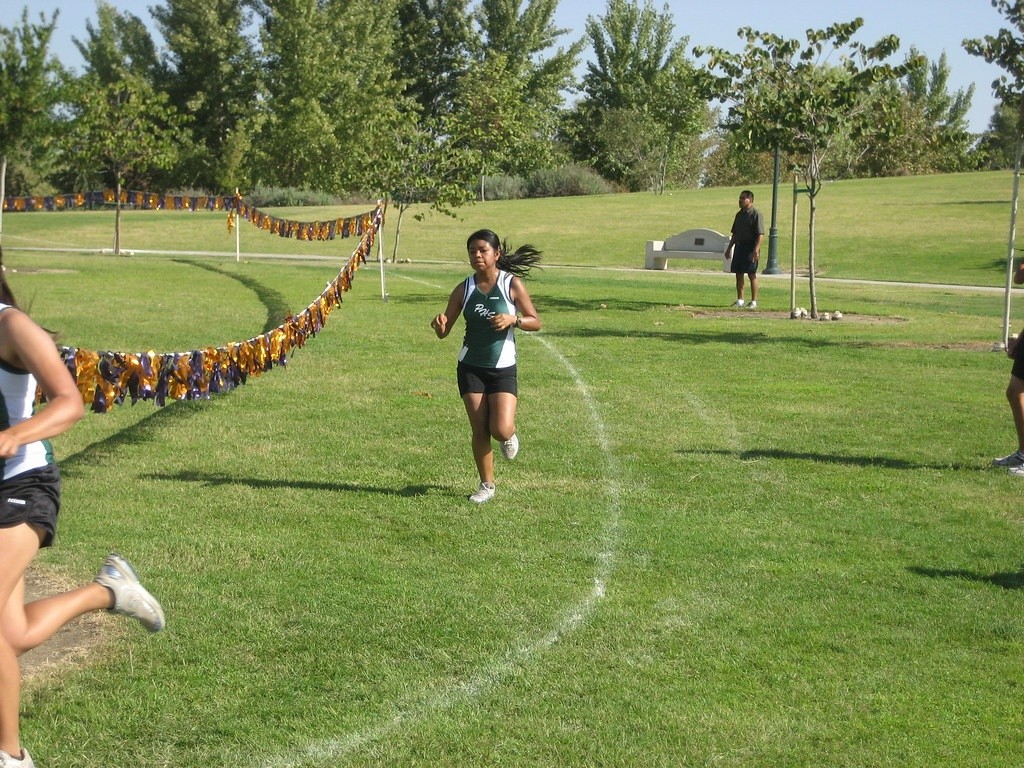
[93,554,164,633]
[500,434,519,460]
[745,301,757,309]
[0,745,36,768]
[1009,462,1024,476]
[731,301,745,307]
[469,480,495,504]
[993,449,1024,466]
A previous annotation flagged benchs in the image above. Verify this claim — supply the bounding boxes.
[644,228,733,273]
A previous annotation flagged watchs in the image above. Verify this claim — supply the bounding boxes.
[513,316,521,328]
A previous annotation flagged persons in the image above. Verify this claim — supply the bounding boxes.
[993,258,1024,476]
[0,248,166,768]
[725,190,765,308]
[431,229,540,503]
